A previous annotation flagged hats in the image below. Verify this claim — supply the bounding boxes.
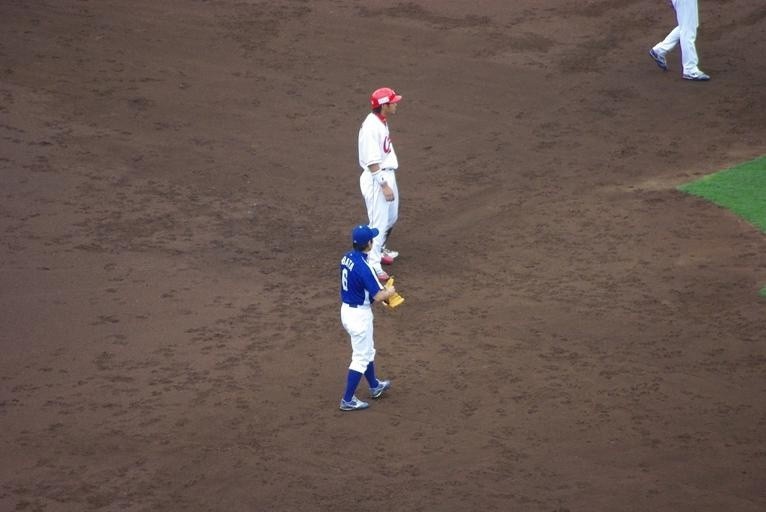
[352,225,379,245]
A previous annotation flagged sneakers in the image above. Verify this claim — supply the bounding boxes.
[376,269,390,281]
[382,248,399,258]
[649,49,668,71]
[682,71,710,80]
[371,379,391,398]
[339,395,370,411]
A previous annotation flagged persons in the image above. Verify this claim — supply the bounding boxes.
[339,225,396,412]
[648,0,710,81]
[357,86,402,281]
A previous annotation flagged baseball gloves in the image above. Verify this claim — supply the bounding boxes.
[382,278,404,308]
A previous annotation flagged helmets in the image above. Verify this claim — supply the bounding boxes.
[370,87,403,110]
